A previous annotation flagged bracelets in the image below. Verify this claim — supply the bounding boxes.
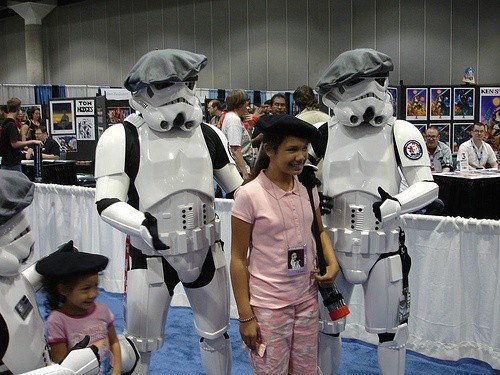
[239,315,255,322]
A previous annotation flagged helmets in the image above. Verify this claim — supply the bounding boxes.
[323,75,395,126]
[128,74,203,131]
[0,210,35,278]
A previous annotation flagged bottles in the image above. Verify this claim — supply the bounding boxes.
[460,152,468,176]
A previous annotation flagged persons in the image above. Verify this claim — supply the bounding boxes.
[0,168,101,375]
[35,240,121,375]
[94,48,245,375]
[221,89,254,185]
[454,123,498,173]
[298,48,439,374]
[420,127,453,172]
[25,126,59,160]
[290,252,300,269]
[208,100,224,126]
[293,85,332,130]
[22,107,44,150]
[230,115,340,375]
[0,97,43,171]
[241,94,288,136]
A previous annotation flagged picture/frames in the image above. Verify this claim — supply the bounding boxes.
[48,98,75,136]
[402,84,481,157]
[106,106,132,125]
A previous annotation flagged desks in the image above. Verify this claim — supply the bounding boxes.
[431,170,500,221]
[23,160,79,186]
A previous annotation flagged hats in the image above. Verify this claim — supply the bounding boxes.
[124,47,208,92]
[0,169,35,227]
[256,114,320,145]
[36,251,108,278]
[314,48,394,97]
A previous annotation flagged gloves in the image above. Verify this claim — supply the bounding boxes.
[141,211,170,251]
[58,240,77,250]
[59,334,101,375]
[315,177,333,216]
[372,186,400,222]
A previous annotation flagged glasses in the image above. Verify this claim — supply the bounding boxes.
[425,133,440,140]
